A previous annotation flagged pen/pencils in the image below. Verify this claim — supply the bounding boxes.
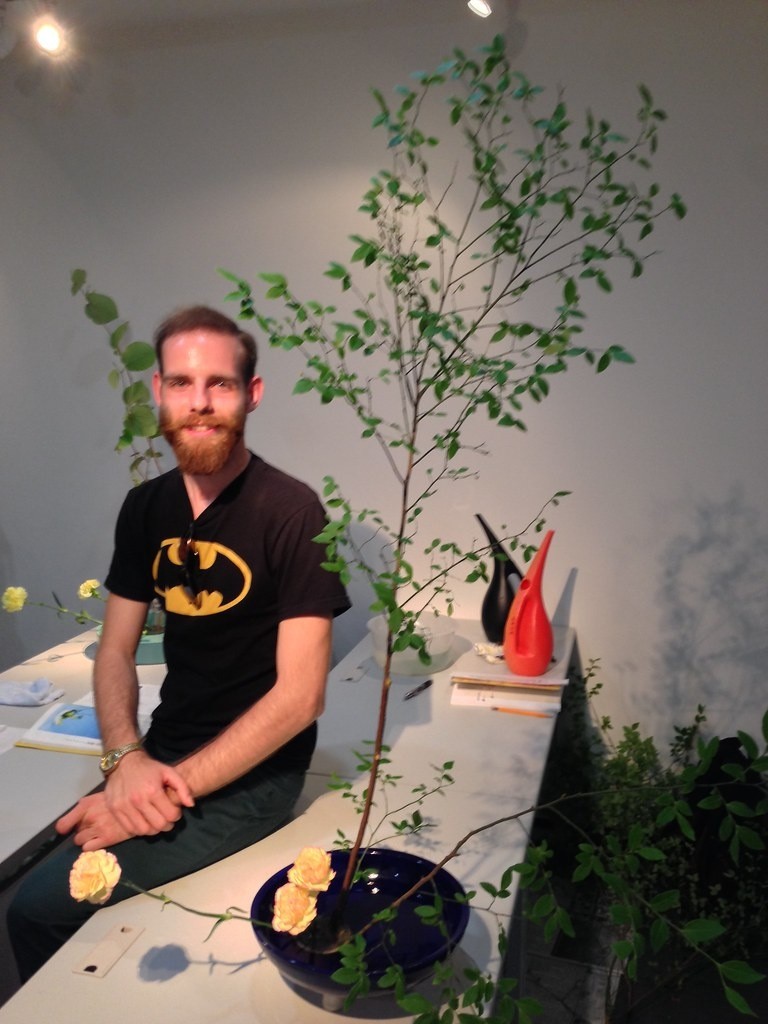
[404,679,433,700]
[492,706,554,718]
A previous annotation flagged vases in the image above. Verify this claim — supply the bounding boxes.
[96,622,166,664]
[250,849,470,1019]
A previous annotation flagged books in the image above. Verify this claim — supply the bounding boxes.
[447,670,570,713]
[14,683,164,757]
[547,874,636,976]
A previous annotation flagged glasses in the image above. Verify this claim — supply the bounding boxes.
[178,523,203,610]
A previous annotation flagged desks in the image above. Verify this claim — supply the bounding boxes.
[0,623,168,865]
[0,611,576,1024]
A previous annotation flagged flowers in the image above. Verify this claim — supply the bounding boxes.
[68,37,768,1024]
[3,269,160,625]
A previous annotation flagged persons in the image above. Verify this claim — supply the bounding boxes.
[5,305,355,987]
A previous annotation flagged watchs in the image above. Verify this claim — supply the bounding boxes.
[98,741,147,783]
